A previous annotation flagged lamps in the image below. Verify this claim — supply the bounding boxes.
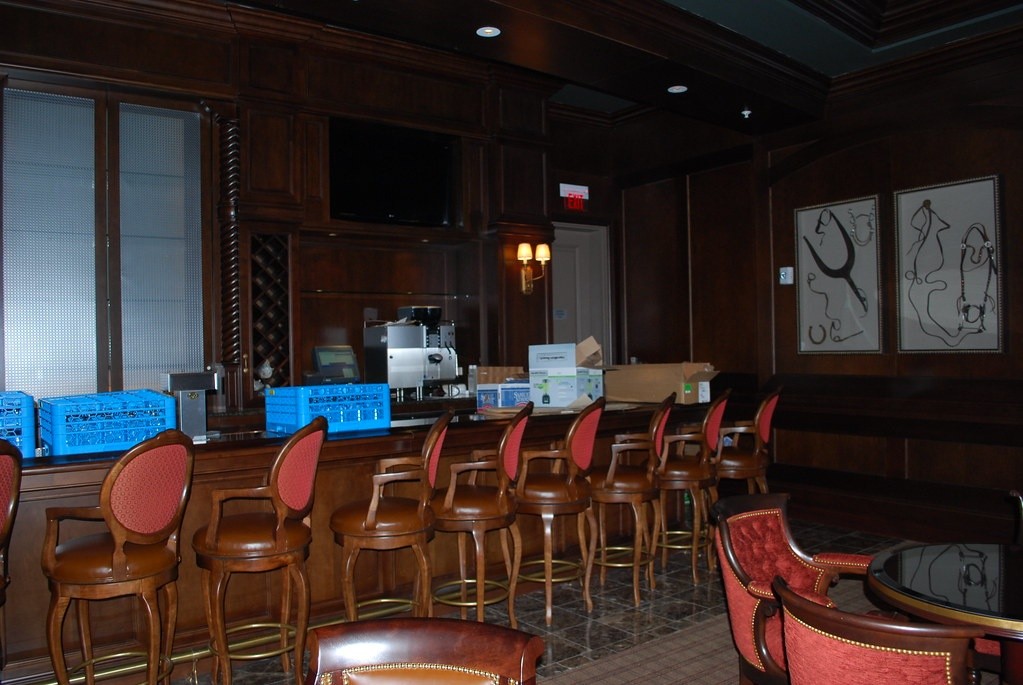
[515,242,552,296]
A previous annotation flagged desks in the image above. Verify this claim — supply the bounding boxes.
[862,541,1023,685]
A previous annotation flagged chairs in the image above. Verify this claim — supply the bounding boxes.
[579,391,679,606]
[429,400,534,630]
[191,415,329,685]
[504,395,607,624]
[302,615,547,685]
[710,490,1004,685]
[0,436,22,675]
[329,412,455,624]
[40,429,196,685]
[640,386,734,586]
[707,392,779,511]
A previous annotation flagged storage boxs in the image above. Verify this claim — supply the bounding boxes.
[603,361,721,407]
[38,387,177,467]
[527,344,604,410]
[477,383,530,409]
[265,383,393,438]
